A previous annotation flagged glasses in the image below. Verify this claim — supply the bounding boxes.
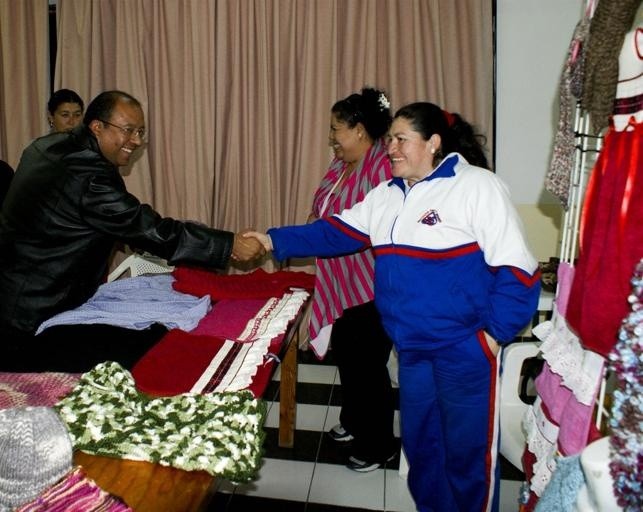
[100,118,145,138]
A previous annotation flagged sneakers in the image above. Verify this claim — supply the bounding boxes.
[346,453,398,472]
[330,423,354,441]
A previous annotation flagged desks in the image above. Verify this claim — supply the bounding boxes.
[0,273,313,511]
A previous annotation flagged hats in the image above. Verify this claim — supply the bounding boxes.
[0,407,74,506]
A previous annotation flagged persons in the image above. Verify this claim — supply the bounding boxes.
[304,85,398,473]
[0,90,266,371]
[44,88,84,134]
[228,100,541,511]
